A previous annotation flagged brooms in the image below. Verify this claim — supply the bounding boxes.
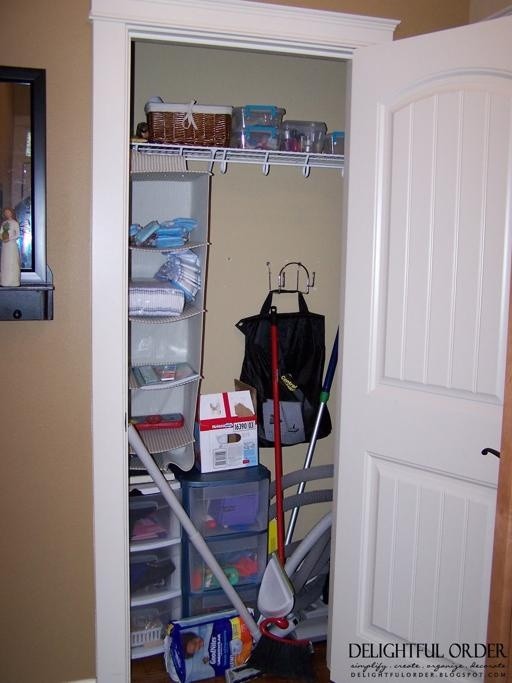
[246,307,318,683]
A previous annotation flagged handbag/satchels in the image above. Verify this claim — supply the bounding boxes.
[239,290,331,448]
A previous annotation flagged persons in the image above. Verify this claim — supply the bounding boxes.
[1,206,22,289]
[176,631,211,665]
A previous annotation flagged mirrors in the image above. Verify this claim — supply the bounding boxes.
[0,65,54,321]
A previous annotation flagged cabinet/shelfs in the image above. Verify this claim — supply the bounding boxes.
[93,3,512,683]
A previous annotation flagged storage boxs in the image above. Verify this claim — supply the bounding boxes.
[130,377,270,660]
[232,105,343,155]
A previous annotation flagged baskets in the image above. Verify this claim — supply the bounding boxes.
[145,102,233,145]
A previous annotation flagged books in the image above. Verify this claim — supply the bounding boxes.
[131,361,199,390]
